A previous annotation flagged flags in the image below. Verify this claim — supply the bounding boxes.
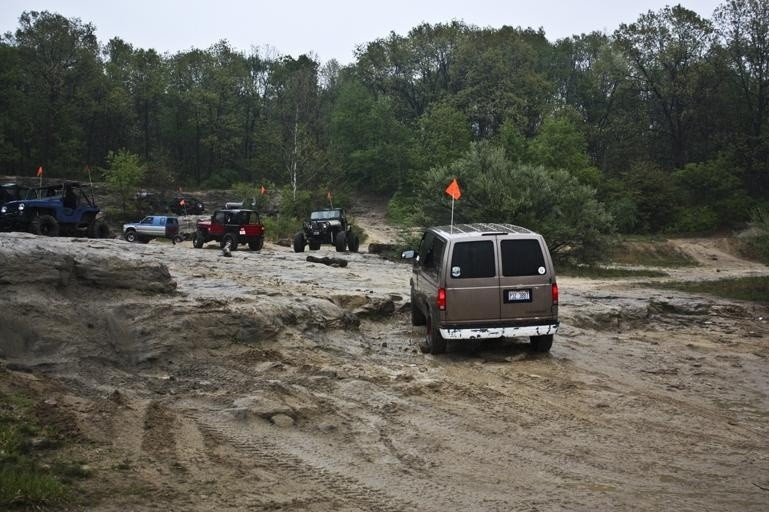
[259,183,266,194]
[180,200,184,206]
[37,167,43,176]
[445,179,461,200]
[327,192,334,199]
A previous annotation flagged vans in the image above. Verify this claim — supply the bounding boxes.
[0,181,38,233]
[399,220,561,357]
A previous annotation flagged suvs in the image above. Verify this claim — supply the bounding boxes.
[1,181,104,240]
[291,207,360,253]
[170,193,205,216]
[119,212,180,242]
[192,207,266,253]
